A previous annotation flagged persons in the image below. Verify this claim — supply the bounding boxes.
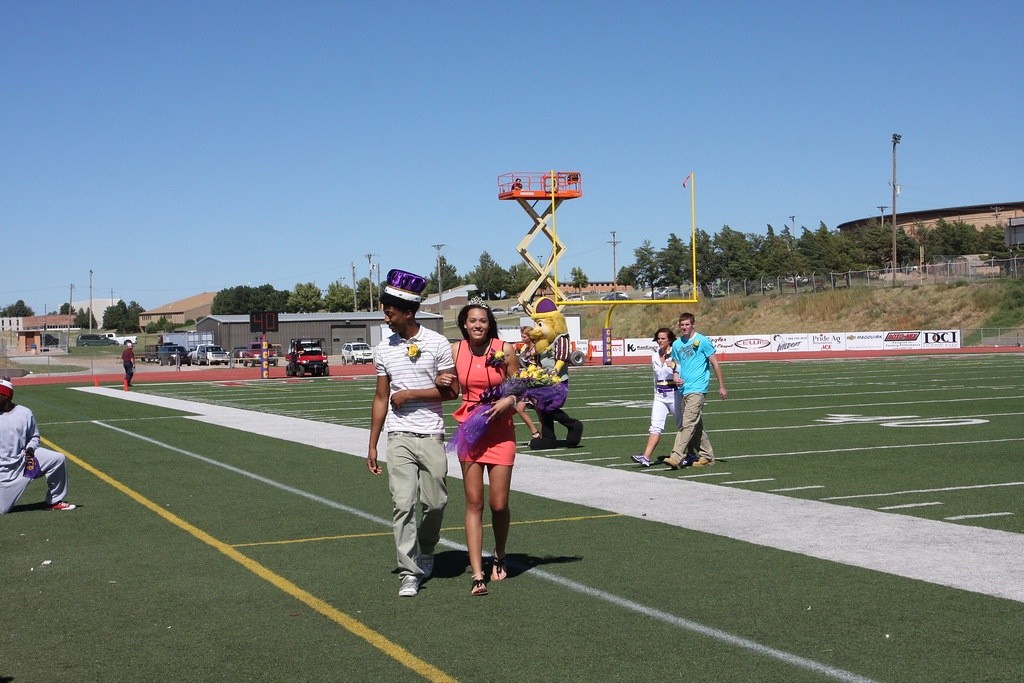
[366,270,460,597]
[121,342,135,387]
[0,376,75,514]
[657,312,727,469]
[511,178,522,190]
[436,297,518,595]
[515,325,543,444]
[632,328,698,467]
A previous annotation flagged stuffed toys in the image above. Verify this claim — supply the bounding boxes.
[526,297,584,450]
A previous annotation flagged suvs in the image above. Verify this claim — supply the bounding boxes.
[341,341,375,365]
[197,345,228,366]
[285,337,329,378]
[76,334,119,346]
[157,346,192,366]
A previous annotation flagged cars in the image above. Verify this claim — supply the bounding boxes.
[565,294,586,301]
[785,266,880,292]
[644,282,725,300]
[491,301,535,319]
[600,291,629,301]
[117,342,284,365]
[243,342,278,367]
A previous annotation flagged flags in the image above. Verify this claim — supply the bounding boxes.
[683,176,689,188]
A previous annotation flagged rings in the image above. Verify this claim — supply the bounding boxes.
[446,381,448,384]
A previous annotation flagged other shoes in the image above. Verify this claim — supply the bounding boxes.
[528,431,542,446]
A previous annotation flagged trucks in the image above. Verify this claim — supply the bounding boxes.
[101,333,137,345]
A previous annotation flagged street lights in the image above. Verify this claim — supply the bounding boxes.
[432,243,444,315]
[366,254,374,312]
[891,133,902,288]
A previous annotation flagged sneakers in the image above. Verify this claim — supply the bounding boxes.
[692,456,715,467]
[51,501,77,511]
[631,454,650,467]
[418,552,434,578]
[682,452,699,466]
[399,575,418,597]
[663,455,682,470]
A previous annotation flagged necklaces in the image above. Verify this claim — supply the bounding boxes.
[471,355,485,368]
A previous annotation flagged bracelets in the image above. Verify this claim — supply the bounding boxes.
[509,395,517,407]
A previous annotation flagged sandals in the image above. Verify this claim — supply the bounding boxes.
[490,547,507,582]
[471,575,488,596]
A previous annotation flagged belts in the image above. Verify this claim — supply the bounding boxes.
[388,431,444,439]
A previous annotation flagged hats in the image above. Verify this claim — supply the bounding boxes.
[0,376,13,399]
[385,269,427,302]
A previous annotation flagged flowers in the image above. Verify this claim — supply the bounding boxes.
[692,340,700,349]
[664,350,671,359]
[406,344,420,359]
[446,356,569,454]
[484,351,507,366]
